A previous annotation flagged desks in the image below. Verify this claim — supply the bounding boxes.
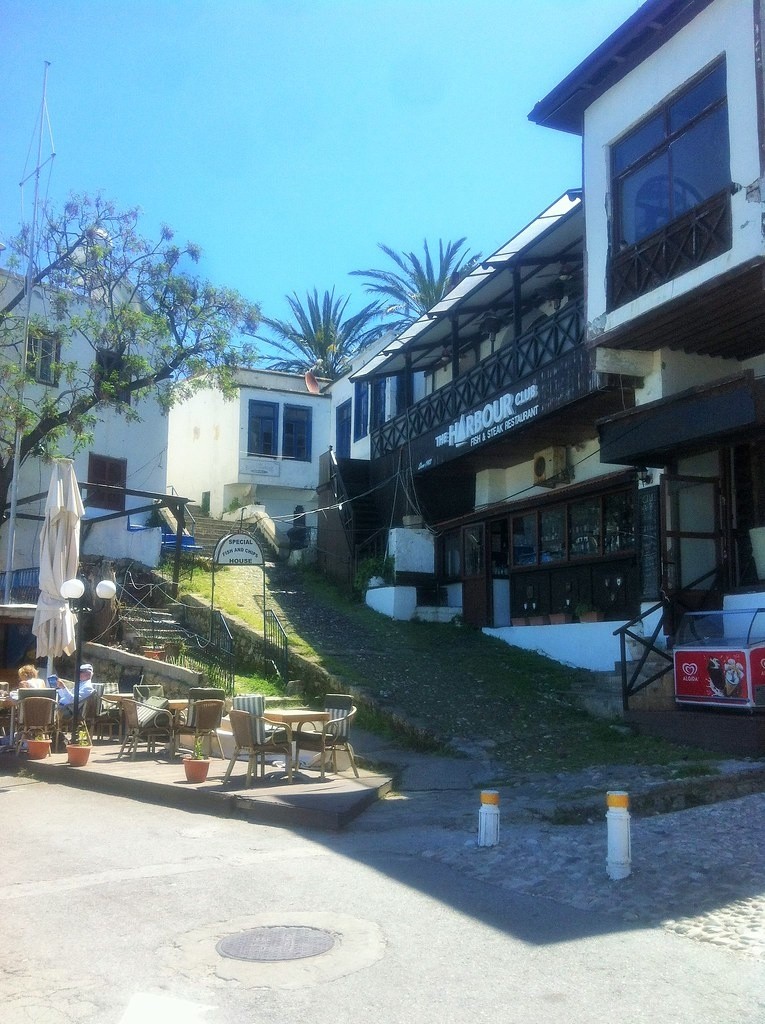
[0,696,20,753]
[263,709,329,785]
[166,698,193,757]
[103,693,135,745]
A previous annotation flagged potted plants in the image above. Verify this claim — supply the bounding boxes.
[66,728,92,766]
[574,600,608,623]
[527,608,551,626]
[510,608,529,626]
[549,604,574,624]
[183,734,211,783]
[165,638,185,661]
[28,734,53,759]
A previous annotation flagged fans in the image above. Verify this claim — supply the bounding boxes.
[425,343,467,370]
[540,262,583,284]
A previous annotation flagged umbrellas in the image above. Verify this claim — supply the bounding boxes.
[32,458,85,687]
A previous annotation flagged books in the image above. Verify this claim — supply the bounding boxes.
[47,675,59,689]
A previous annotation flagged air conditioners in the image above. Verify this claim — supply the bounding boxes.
[534,446,567,484]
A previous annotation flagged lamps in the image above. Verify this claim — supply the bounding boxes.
[636,465,653,486]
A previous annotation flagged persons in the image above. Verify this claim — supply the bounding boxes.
[18,664,46,724]
[56,664,94,718]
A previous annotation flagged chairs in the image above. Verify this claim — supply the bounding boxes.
[133,684,169,755]
[117,698,173,760]
[171,689,226,761]
[294,693,359,781]
[13,688,56,757]
[223,694,293,788]
[57,680,123,745]
[0,682,9,737]
